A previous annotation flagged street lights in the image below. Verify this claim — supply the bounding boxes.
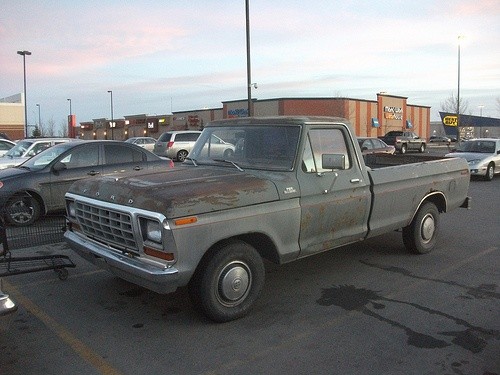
[107,90,115,140]
[36,104,40,130]
[456,34,466,140]
[17,50,32,138]
[67,98,72,115]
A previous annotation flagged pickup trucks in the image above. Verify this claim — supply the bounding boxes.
[60,115,472,325]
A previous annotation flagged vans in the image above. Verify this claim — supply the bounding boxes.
[152,130,236,162]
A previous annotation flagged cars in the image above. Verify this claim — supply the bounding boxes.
[444,137,500,182]
[124,137,158,153]
[0,132,96,169]
[378,130,427,153]
[355,137,396,155]
[430,135,456,143]
[0,139,176,228]
